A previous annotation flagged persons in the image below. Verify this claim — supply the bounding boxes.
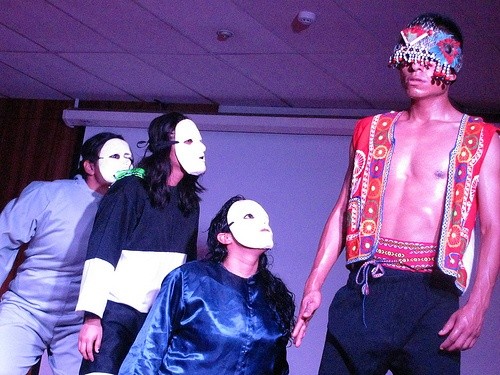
[75,111,209,375]
[0,132,135,375]
[292,11,500,375]
[118,194,297,375]
